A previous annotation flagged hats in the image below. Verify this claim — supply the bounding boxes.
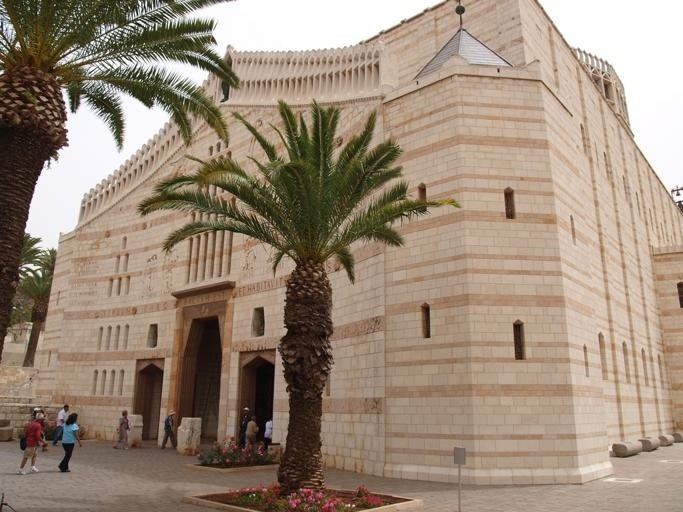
[169,410,176,416]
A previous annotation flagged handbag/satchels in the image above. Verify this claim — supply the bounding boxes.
[20,438,26,450]
[54,426,63,441]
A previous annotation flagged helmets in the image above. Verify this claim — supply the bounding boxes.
[244,407,249,411]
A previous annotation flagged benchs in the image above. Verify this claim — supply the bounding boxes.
[611,432,683,457]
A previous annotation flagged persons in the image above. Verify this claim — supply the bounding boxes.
[160,408,177,450]
[238,407,249,449]
[111,410,131,450]
[16,413,47,474]
[51,404,70,446]
[30,406,49,451]
[244,414,259,449]
[262,416,272,449]
[56,413,83,473]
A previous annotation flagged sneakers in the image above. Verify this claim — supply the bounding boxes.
[18,467,26,474]
[31,468,39,472]
